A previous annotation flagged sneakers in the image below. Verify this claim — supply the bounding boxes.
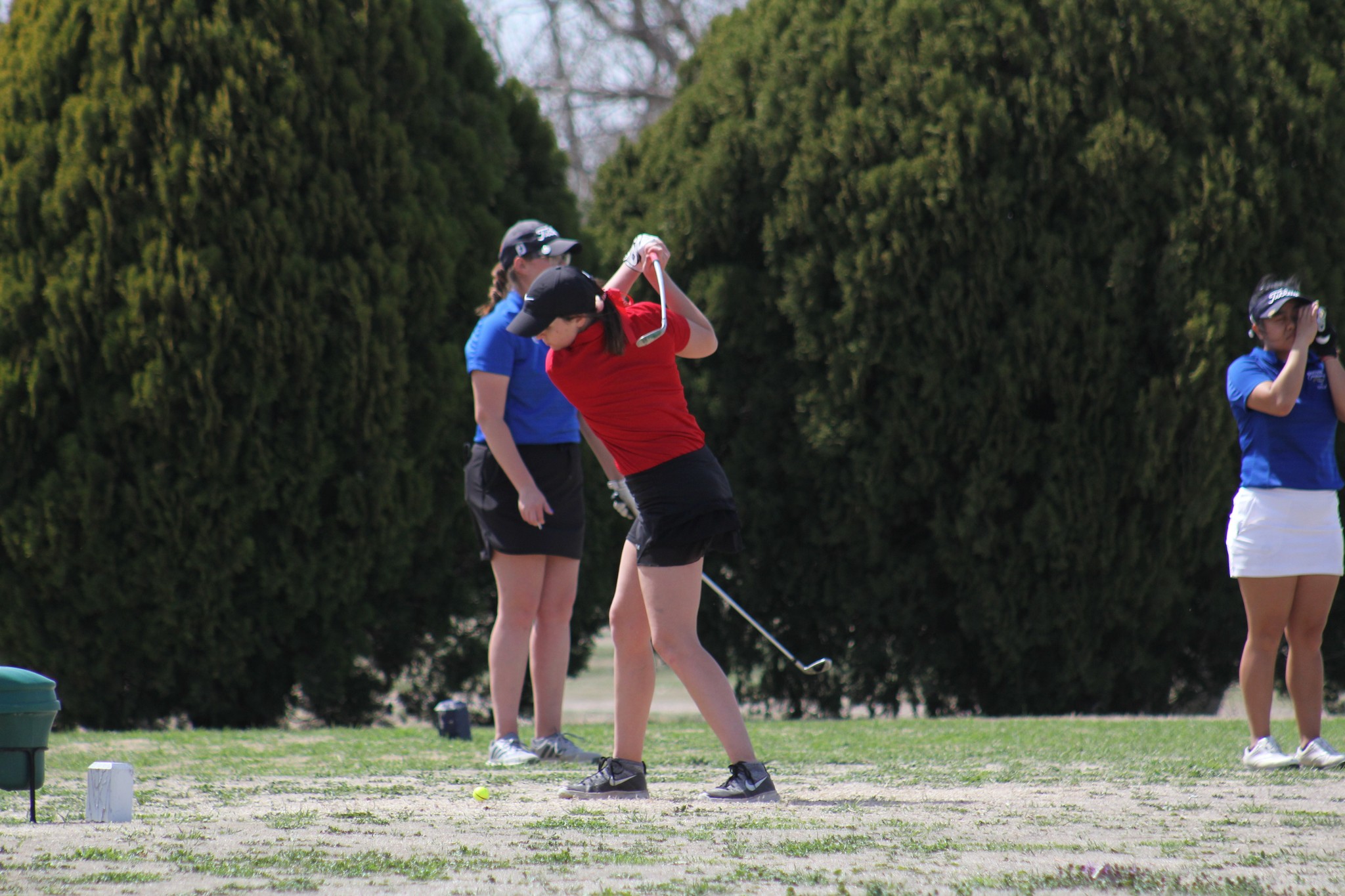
[530,732,602,762]
[1294,736,1345,769]
[485,731,540,766]
[557,757,650,799]
[1243,736,1300,770]
[698,760,780,803]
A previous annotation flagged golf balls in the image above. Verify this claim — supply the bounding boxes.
[474,786,489,801]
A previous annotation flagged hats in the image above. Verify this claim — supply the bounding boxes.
[1249,287,1313,323]
[498,221,583,273]
[505,266,608,337]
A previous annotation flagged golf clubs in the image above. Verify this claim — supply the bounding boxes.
[635,251,668,349]
[611,489,833,676]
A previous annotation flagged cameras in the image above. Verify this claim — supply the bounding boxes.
[1292,304,1328,335]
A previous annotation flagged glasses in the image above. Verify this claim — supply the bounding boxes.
[535,253,571,267]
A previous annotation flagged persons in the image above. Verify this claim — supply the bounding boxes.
[463,220,640,766]
[1225,278,1344,769]
[507,233,783,803]
